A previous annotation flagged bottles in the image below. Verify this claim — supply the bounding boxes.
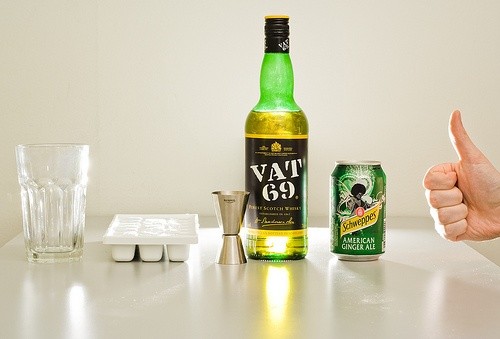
[245,16,309,260]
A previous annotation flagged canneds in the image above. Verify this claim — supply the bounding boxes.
[331,160,386,261]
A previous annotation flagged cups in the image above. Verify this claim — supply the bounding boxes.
[16,143,90,262]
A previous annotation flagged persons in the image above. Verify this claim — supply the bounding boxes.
[421,108,499,244]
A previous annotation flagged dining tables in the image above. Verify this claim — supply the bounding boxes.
[0,216,500,339]
